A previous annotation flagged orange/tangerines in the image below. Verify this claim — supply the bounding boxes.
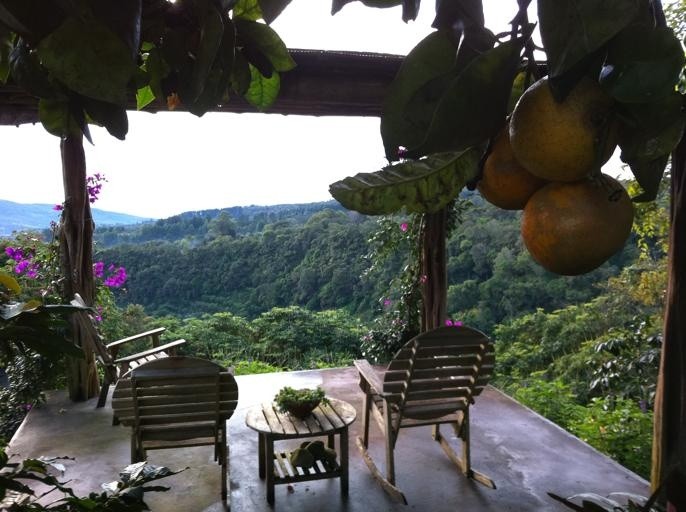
[521,173,635,276]
[476,143,547,211]
[509,74,617,182]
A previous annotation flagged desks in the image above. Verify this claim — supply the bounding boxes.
[245,396,358,507]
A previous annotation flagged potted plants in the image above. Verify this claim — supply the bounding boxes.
[273,386,330,419]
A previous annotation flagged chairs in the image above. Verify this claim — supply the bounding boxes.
[112,355,238,512]
[70,292,187,426]
[353,325,496,506]
